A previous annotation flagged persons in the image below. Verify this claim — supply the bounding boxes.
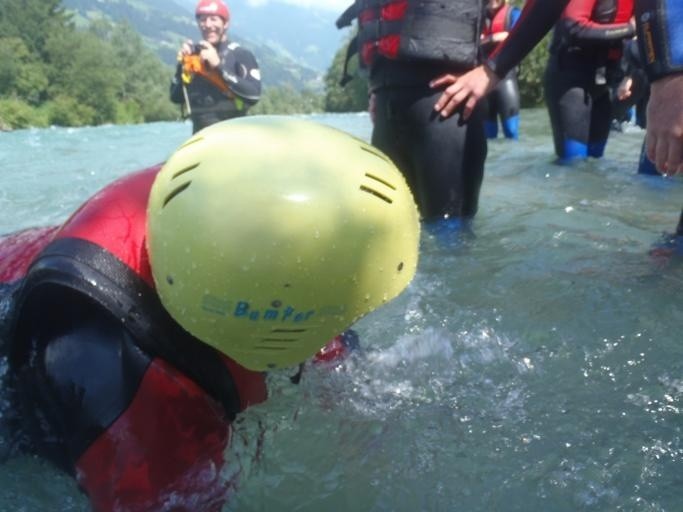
[338,2,522,235]
[429,1,683,242]
[170,1,262,134]
[1,114,422,512]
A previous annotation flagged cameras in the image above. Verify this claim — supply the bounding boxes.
[191,44,207,54]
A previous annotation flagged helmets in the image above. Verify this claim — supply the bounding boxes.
[144,115,423,374]
[196,0,229,24]
